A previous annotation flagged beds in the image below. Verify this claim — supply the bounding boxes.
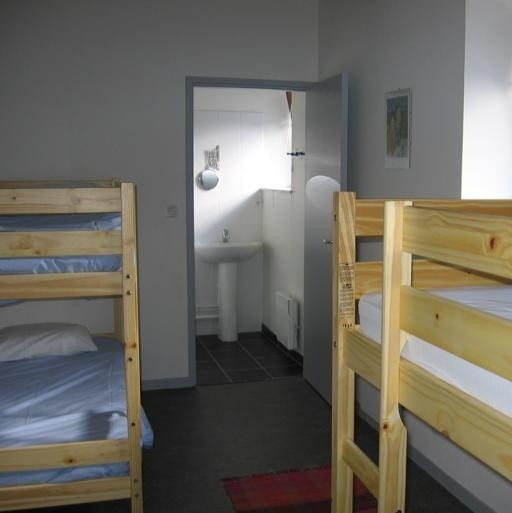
[0,178,142,513]
[331,188,512,512]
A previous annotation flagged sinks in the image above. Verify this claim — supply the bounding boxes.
[193,240,262,260]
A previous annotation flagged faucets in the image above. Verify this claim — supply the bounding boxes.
[221,228,233,241]
